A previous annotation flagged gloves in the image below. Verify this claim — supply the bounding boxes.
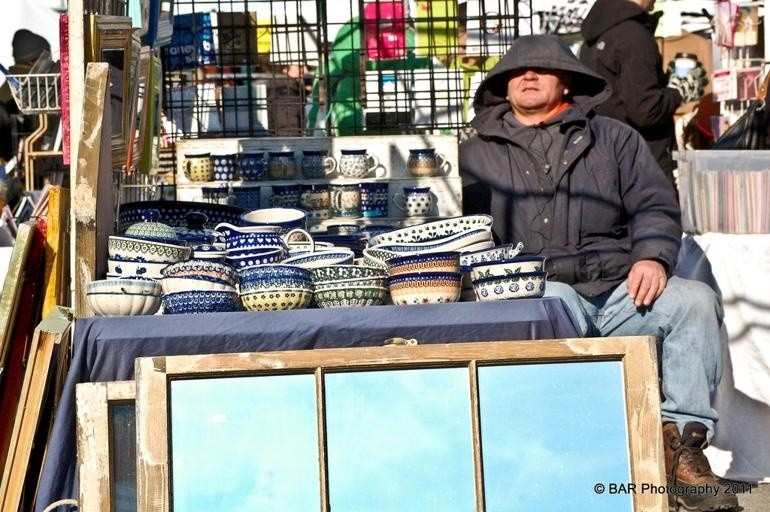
[667,59,709,105]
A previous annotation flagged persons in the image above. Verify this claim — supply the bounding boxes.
[439,20,501,122]
[580,0,707,208]
[0,30,51,159]
[460,34,739,512]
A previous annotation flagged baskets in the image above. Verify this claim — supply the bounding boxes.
[7,73,61,114]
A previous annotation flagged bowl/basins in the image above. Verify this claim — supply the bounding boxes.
[85,207,549,318]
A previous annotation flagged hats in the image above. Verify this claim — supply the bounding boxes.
[12,29,50,63]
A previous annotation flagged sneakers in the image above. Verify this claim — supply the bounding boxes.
[662,422,739,511]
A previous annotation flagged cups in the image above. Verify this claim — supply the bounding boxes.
[182,149,447,218]
[674,52,699,76]
